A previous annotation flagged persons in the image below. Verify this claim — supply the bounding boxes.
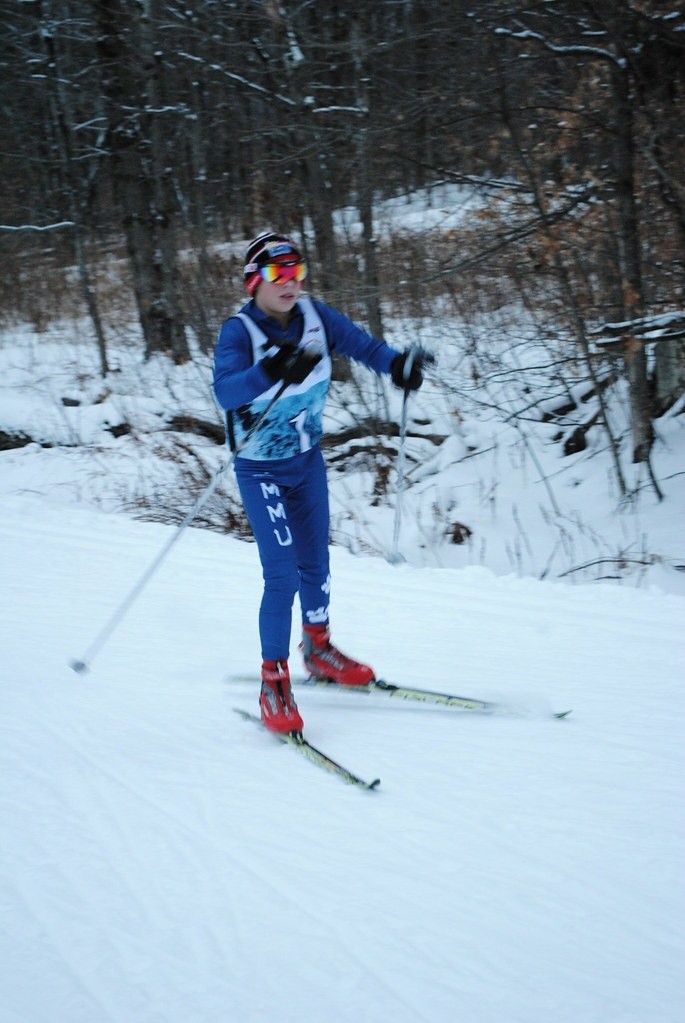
[214,231,433,732]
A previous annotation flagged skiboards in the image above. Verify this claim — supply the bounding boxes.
[228,671,575,792]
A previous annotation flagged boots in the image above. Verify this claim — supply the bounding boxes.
[298,625,374,686]
[260,661,303,733]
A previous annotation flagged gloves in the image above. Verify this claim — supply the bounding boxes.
[389,347,436,391]
[260,338,307,386]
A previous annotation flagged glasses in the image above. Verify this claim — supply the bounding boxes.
[255,257,309,283]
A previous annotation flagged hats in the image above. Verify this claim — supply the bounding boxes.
[243,232,304,296]
